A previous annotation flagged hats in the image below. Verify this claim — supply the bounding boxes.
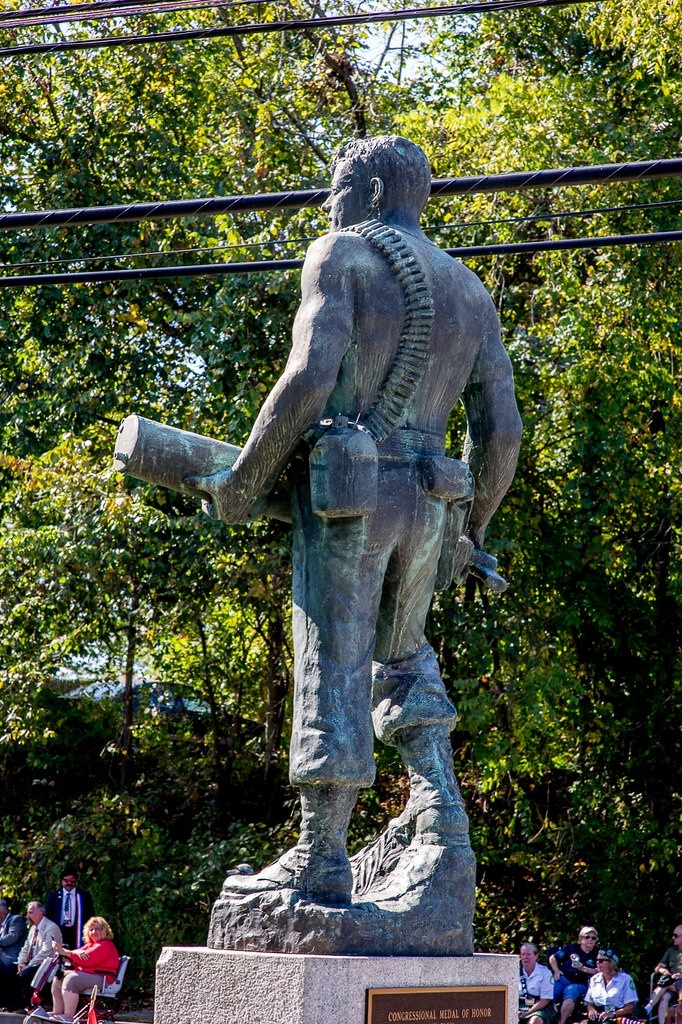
[598,946,620,964]
[579,926,598,935]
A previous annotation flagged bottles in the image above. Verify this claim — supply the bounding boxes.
[569,953,581,966]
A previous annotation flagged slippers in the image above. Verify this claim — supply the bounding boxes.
[46,1012,73,1023]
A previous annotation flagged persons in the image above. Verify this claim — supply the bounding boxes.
[0,871,122,1023]
[516,925,682,1024]
[182,133,526,907]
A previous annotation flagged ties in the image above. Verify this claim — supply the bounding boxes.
[63,893,71,925]
[29,929,39,962]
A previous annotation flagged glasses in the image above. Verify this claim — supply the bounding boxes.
[596,959,611,963]
[672,933,682,938]
[584,936,597,940]
[63,878,76,883]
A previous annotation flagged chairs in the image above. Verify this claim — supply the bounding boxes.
[72,955,131,1024]
[546,947,680,1024]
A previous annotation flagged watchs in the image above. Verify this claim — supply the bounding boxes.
[612,1011,615,1017]
[578,963,584,971]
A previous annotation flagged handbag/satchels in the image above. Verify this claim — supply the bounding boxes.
[657,974,675,987]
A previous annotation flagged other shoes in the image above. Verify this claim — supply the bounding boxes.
[636,1001,650,1024]
